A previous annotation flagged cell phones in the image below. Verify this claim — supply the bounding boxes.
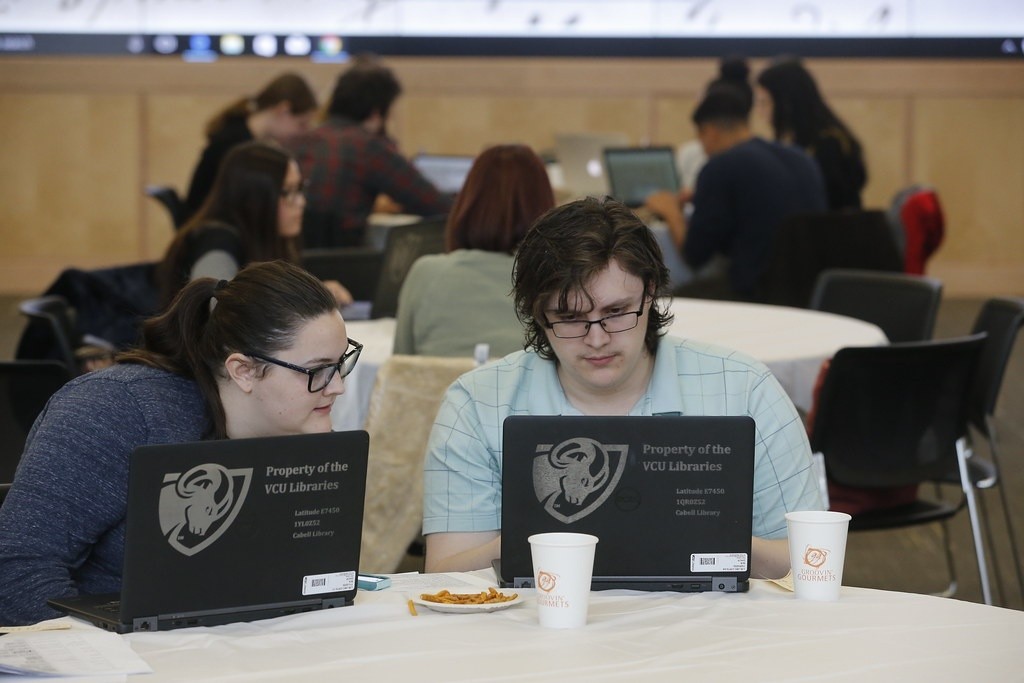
[357,573,391,591]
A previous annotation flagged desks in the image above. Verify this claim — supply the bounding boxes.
[0,572,1024,683]
[366,210,696,297]
[331,296,890,435]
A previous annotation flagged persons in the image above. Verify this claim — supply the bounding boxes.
[285,63,451,245]
[646,88,826,303]
[395,144,555,356]
[0,259,362,627]
[183,74,318,219]
[420,197,824,580]
[758,62,868,211]
[160,143,354,315]
[680,61,755,213]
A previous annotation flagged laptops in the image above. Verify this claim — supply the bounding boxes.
[410,153,475,194]
[603,144,680,209]
[490,415,756,593]
[44,430,370,634]
[340,218,449,321]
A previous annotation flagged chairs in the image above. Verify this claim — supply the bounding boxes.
[363,353,496,577]
[0,187,379,509]
[801,184,1024,613]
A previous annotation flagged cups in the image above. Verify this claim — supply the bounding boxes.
[784,510,852,603]
[527,532,599,629]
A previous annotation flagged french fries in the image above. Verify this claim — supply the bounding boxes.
[409,588,518,616]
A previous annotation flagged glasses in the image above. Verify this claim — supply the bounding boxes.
[541,277,648,339]
[277,178,311,206]
[244,337,363,393]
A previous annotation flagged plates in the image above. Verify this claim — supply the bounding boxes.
[413,595,527,614]
